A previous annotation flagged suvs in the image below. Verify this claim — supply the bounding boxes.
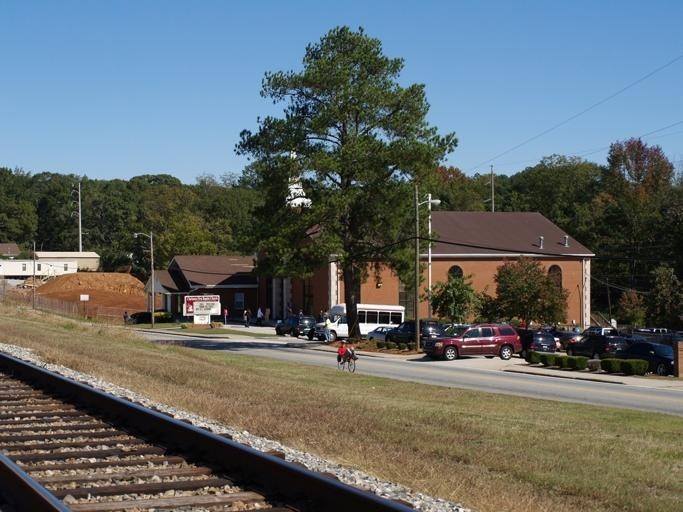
[274,314,316,342]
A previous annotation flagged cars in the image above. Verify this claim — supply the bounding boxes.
[5,256,15,260]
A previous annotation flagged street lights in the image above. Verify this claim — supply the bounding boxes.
[69,182,82,252]
[132,230,154,328]
[26,238,36,311]
[414,183,441,351]
[40,239,49,251]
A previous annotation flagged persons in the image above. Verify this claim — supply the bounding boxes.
[337,340,348,370]
[256,303,264,326]
[286,302,326,322]
[124,312,128,324]
[243,306,249,327]
[223,306,229,325]
